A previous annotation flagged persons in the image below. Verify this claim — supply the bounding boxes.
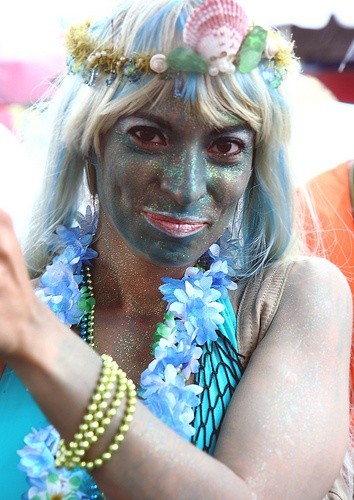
[0,0,353,498]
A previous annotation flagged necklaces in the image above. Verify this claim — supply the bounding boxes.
[84,263,99,348]
[77,244,87,345]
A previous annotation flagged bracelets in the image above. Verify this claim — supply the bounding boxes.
[54,351,137,474]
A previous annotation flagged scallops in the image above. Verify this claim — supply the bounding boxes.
[182,0,251,70]
